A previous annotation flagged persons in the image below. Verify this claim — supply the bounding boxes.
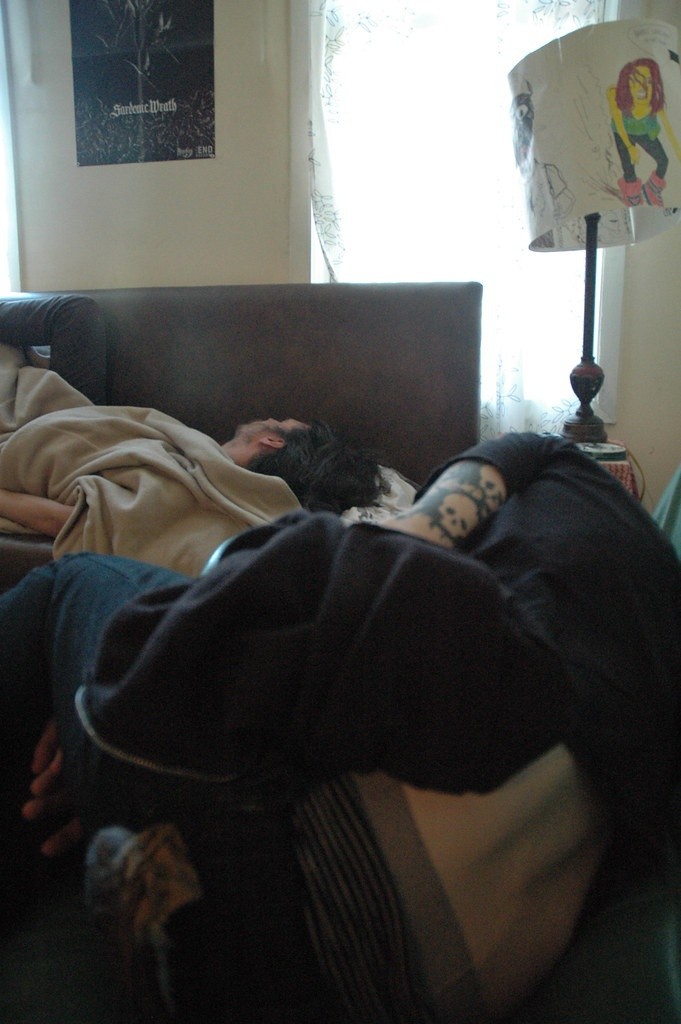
[0,435,681,1024]
[0,344,385,565]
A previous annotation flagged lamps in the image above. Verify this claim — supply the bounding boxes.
[506,19,681,460]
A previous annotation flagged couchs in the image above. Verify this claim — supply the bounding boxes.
[0,283,483,520]
